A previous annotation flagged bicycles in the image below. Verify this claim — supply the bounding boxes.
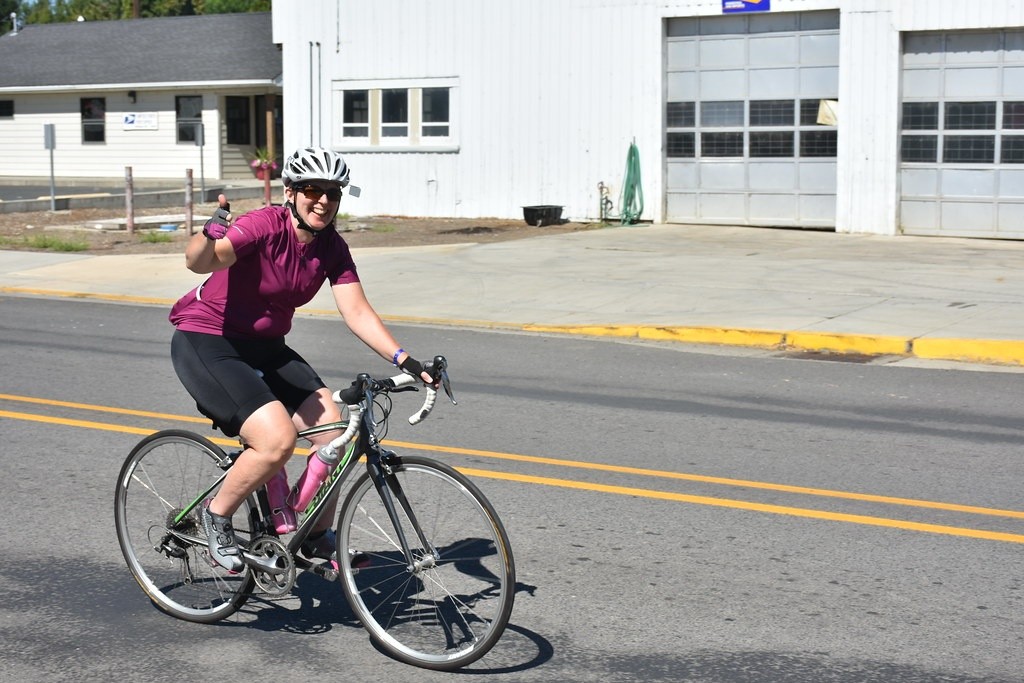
[113,355,517,672]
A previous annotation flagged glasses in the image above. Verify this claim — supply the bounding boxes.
[296,187,342,201]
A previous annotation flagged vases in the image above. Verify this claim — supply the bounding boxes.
[256,166,273,180]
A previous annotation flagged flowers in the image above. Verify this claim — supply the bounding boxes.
[247,145,282,171]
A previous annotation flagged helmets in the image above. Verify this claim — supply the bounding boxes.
[280,146,350,188]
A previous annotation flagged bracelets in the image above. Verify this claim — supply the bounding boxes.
[392,350,404,366]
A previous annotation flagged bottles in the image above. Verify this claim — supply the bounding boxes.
[285,446,339,513]
[266,466,300,534]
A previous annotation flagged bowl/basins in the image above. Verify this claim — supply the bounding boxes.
[160,224,177,231]
[521,205,565,226]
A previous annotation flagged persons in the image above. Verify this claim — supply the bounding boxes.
[169,146,441,574]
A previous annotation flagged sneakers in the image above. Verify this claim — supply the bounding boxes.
[196,498,245,573]
[301,528,372,571]
